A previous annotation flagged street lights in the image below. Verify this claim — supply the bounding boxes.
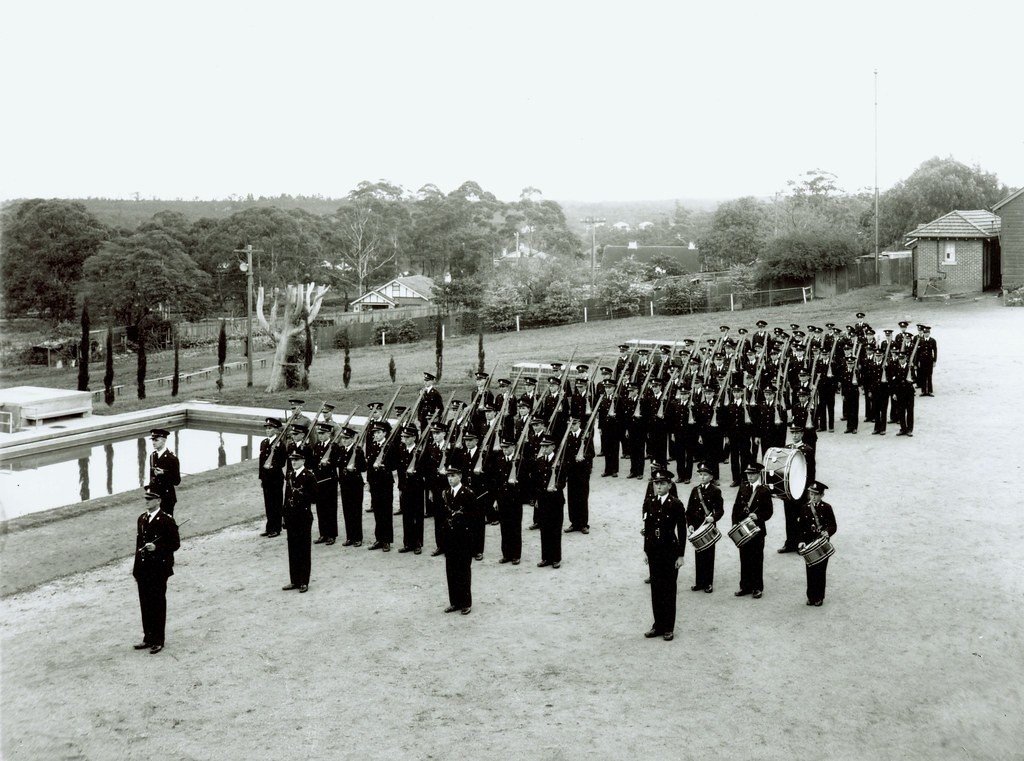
[233,244,266,386]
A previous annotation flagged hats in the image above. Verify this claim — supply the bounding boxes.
[144,486,162,500]
[258,312,940,505]
[150,428,170,439]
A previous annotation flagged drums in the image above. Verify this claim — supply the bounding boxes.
[727,516,761,548]
[762,447,808,501]
[688,522,722,554]
[799,536,837,567]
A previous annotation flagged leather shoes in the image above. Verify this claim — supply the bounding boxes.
[151,644,162,653]
[807,598,815,605]
[664,631,673,640]
[299,584,308,592]
[260,507,590,569]
[644,627,663,637]
[692,584,703,591]
[752,588,762,598]
[704,584,713,593]
[282,583,297,590]
[815,598,823,606]
[734,588,751,596]
[462,606,471,614]
[777,545,794,553]
[444,605,462,613]
[134,642,153,650]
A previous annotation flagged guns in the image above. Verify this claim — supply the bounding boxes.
[263,324,924,492]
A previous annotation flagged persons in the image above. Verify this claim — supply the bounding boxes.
[134,484,180,655]
[258,312,938,641]
[149,429,181,567]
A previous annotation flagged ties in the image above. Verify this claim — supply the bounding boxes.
[147,515,151,522]
